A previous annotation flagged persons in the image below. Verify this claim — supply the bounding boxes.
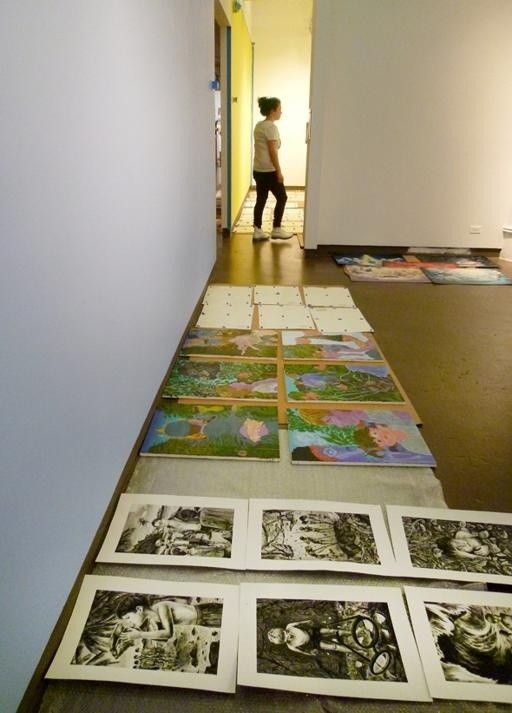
[151,517,203,531]
[266,619,355,655]
[252,96,293,241]
[437,528,490,561]
[115,592,221,643]
[298,510,344,556]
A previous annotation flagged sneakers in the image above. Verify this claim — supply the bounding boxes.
[270,227,294,239]
[252,224,270,240]
